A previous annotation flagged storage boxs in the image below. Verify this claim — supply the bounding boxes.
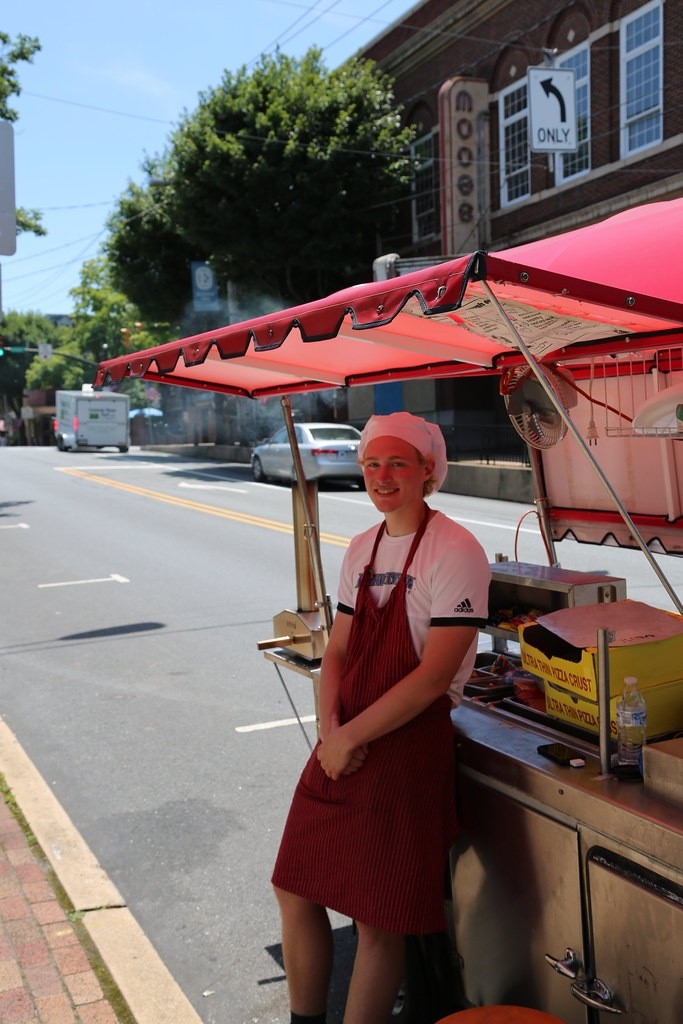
[520,600,683,702]
[642,740,683,811]
[544,679,683,743]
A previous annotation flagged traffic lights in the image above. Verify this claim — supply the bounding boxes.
[120,328,129,346]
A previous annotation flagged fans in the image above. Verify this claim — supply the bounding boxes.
[500,363,568,450]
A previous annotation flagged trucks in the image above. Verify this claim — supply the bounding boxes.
[49,384,130,454]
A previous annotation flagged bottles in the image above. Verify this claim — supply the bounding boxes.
[615,676,647,764]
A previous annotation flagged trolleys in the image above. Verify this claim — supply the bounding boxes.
[96,198,683,1024]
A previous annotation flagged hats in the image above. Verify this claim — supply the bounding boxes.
[357,413,449,497]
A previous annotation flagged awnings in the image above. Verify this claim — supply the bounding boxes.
[92,250,683,637]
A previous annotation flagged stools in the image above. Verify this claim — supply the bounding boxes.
[434,1004,562,1024]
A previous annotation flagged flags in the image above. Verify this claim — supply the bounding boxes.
[146,383,161,401]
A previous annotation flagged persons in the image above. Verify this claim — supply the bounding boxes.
[273,411,492,1024]
[0,417,6,446]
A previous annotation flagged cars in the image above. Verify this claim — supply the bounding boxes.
[250,423,368,489]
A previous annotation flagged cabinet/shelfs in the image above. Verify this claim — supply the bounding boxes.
[438,765,683,1024]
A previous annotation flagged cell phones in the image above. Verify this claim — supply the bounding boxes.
[537,743,586,767]
